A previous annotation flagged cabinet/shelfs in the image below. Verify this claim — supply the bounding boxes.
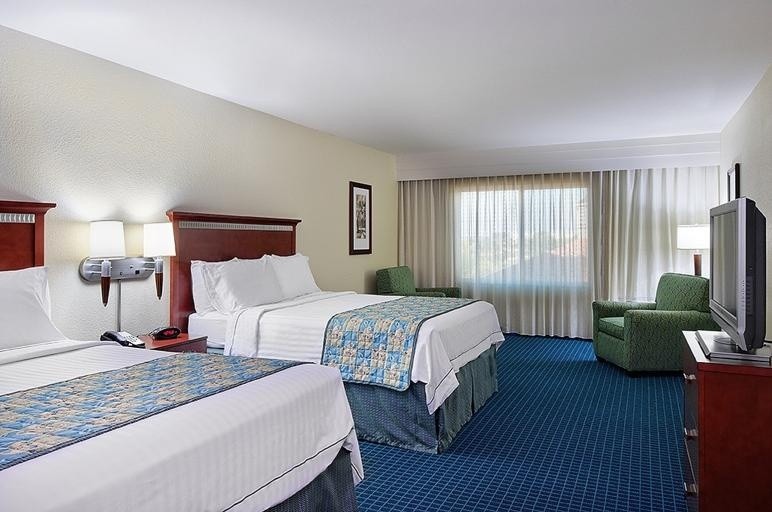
[677,328,771,511]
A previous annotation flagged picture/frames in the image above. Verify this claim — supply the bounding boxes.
[348,181,373,256]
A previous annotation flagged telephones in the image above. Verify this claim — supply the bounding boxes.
[100,331,145,348]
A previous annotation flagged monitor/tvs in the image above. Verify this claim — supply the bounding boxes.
[694,197,772,362]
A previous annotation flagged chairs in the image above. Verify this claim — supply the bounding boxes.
[589,271,722,374]
